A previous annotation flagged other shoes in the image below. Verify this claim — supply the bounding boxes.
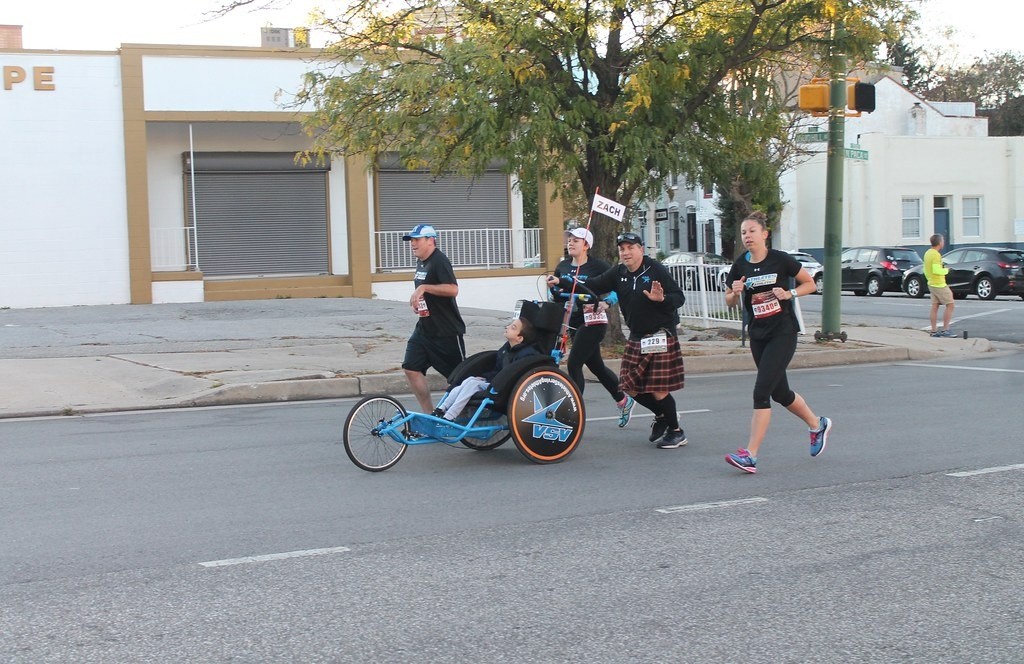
[432,408,443,419]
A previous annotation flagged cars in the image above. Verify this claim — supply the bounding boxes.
[717,252,823,291]
[661,252,734,291]
[901,247,1024,300]
[813,246,924,297]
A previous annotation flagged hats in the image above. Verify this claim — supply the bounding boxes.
[565,226,593,249]
[402,224,436,241]
[615,232,642,246]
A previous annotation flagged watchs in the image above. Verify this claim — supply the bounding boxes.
[789,289,797,297]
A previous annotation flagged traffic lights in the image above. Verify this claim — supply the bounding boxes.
[847,82,876,112]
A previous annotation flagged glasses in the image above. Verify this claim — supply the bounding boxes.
[616,234,637,240]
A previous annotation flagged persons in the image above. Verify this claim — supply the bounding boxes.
[724,210,833,473]
[923,234,958,338]
[402,224,466,413]
[430,316,538,422]
[548,228,637,428]
[546,232,688,448]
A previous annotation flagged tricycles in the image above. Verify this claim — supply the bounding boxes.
[342,270,600,472]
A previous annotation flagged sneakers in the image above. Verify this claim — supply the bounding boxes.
[618,396,636,428]
[930,331,941,337]
[808,416,832,457]
[941,330,957,337]
[725,447,757,473]
[658,428,688,448]
[648,416,669,442]
